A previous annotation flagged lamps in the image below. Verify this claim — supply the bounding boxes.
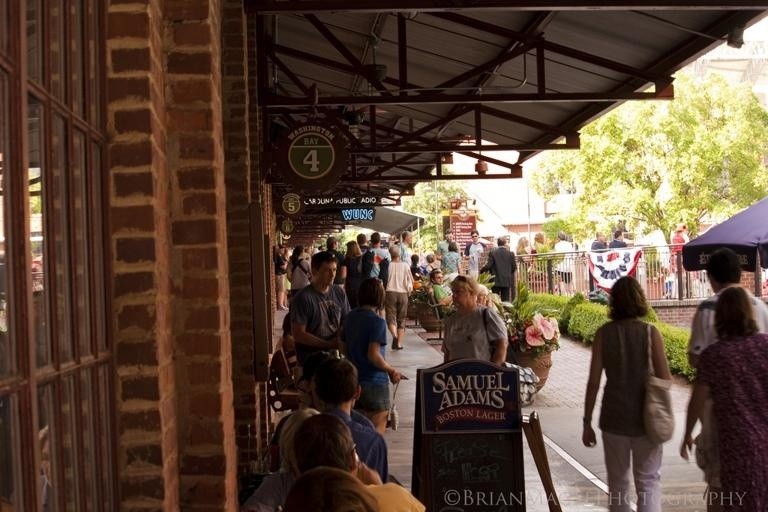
[358,28,389,86]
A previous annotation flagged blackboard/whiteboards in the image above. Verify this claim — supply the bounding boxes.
[413,358,526,512]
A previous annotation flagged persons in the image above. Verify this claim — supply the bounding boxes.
[239,231,767,512]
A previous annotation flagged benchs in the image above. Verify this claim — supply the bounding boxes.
[267,338,303,412]
[426,289,456,344]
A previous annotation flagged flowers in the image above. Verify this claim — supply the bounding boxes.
[411,268,458,304]
[495,279,563,361]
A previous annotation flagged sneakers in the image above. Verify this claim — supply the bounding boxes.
[391,336,403,350]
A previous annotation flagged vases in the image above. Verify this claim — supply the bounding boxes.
[512,348,552,393]
[414,302,440,332]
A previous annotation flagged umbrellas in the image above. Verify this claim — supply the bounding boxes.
[682,196,768,272]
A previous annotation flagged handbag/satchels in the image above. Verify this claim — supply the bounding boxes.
[379,259,390,291]
[644,375,675,445]
[361,251,375,280]
